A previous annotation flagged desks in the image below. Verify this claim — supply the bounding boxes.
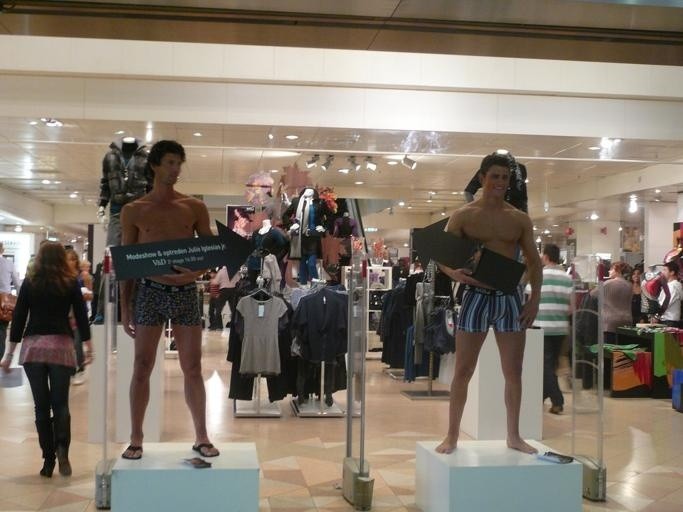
[616,326,683,399]
[582,345,653,398]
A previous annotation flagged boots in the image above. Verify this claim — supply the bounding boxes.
[35,414,73,477]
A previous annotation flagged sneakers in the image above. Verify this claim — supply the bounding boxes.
[549,404,563,413]
[208,323,231,331]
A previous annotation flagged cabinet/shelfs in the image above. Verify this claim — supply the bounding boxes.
[341,266,393,360]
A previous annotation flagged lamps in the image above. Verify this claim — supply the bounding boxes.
[402,154,417,170]
[305,154,320,168]
[363,156,377,172]
[320,155,335,171]
[347,156,361,171]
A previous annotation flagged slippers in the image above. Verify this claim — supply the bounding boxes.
[121,445,143,460]
[193,443,221,458]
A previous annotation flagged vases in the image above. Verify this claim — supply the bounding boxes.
[373,256,383,264]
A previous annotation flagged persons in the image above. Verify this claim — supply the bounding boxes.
[464,148,528,215]
[120,139,219,459]
[434,153,543,453]
[1,242,94,386]
[202,264,240,330]
[98,137,151,246]
[233,207,252,238]
[523,244,577,413]
[248,219,288,278]
[590,261,683,390]
[0,240,94,479]
[281,187,335,285]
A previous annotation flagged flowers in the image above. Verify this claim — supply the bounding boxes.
[371,236,385,258]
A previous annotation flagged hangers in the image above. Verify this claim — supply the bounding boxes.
[248,278,325,297]
